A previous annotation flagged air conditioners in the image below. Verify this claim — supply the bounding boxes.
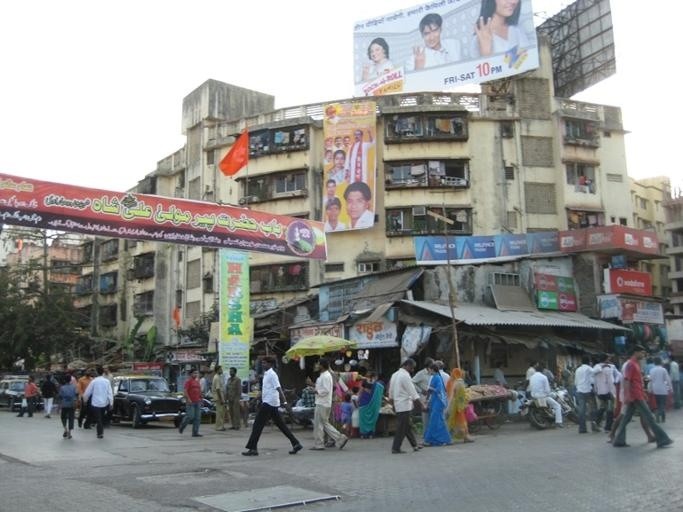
[410,205,426,217]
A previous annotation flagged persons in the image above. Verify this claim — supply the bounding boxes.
[493,361,507,386]
[244,356,303,453]
[178,365,242,437]
[16,374,56,418]
[360,39,392,82]
[301,355,478,453]
[55,367,114,439]
[402,13,460,72]
[526,347,681,448]
[322,127,373,233]
[469,1,530,61]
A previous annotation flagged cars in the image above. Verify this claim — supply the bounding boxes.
[0,379,45,411]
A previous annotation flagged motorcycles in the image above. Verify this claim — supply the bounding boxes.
[515,380,585,429]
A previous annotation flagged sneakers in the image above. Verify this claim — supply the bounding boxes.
[392,449,403,453]
[310,436,348,450]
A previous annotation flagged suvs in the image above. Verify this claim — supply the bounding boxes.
[112,375,188,430]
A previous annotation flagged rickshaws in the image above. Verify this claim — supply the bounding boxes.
[421,389,519,432]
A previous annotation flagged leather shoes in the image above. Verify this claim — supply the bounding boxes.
[289,444,302,454]
[242,449,258,455]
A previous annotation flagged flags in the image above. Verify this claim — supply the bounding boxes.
[216,126,246,177]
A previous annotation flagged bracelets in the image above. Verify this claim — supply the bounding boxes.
[283,402,287,405]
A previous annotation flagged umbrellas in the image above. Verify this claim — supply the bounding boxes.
[286,335,357,361]
